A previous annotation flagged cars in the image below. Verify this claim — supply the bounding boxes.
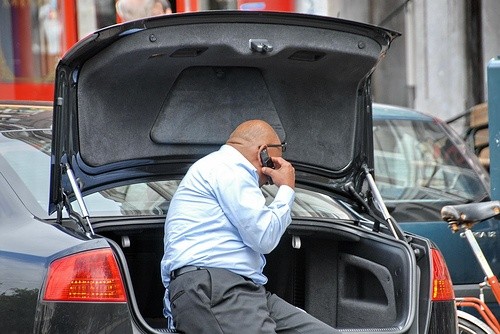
[0,11,459,331]
[372,102,496,322]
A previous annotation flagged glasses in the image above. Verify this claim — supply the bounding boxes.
[258,142,288,152]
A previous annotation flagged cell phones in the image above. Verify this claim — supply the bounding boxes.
[259,145,278,187]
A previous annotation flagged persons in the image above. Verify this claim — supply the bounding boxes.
[159,119,340,334]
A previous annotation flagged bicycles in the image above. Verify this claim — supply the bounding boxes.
[441,201,500,334]
